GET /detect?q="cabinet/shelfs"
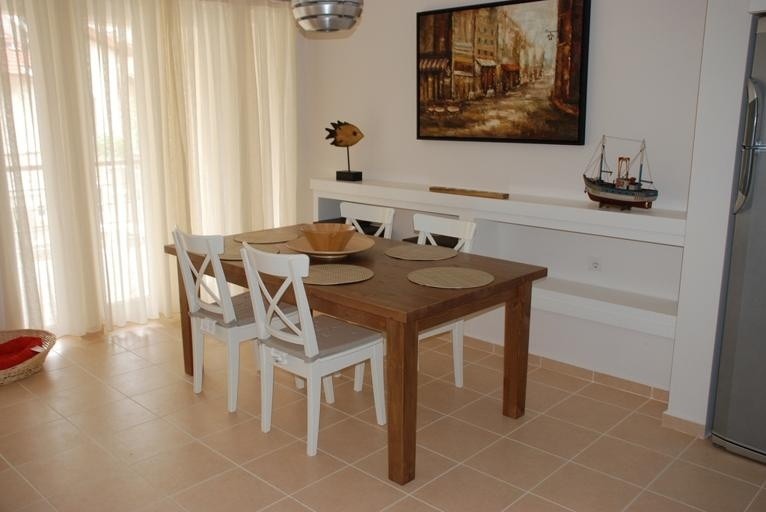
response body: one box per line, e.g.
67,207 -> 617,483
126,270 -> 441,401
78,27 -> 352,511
312,177 -> 686,336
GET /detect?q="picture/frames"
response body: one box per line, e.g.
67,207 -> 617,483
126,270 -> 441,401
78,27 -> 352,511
416,1 -> 593,146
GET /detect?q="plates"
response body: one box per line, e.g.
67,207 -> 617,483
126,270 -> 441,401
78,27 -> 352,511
285,236 -> 375,259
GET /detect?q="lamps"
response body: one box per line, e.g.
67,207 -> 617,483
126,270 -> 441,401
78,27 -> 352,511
292,0 -> 363,34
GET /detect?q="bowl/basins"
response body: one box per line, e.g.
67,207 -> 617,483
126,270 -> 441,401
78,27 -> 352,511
300,223 -> 356,252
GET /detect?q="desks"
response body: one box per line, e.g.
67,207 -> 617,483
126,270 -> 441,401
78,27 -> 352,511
165,225 -> 548,483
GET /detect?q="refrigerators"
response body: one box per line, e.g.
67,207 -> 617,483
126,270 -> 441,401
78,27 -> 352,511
709,14 -> 765,465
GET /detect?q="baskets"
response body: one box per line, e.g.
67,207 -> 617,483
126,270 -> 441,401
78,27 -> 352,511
1,329 -> 57,386
